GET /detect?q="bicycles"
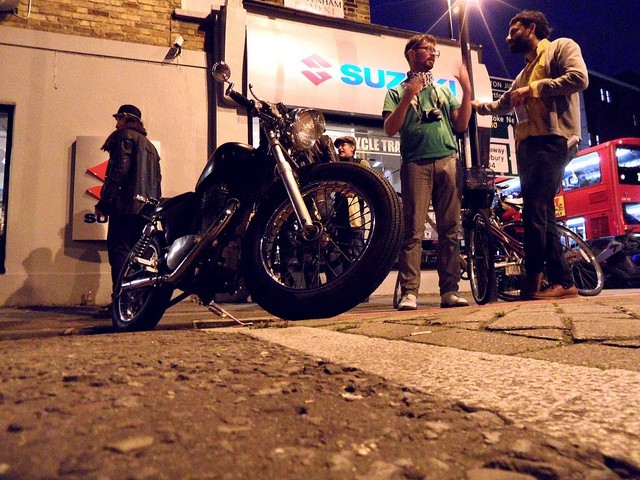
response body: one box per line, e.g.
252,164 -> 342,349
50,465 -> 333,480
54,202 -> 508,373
461,164 -> 603,305
394,191 -> 522,308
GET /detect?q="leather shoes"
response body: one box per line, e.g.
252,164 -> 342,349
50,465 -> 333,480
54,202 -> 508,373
520,272 -> 543,300
440,291 -> 468,307
398,294 -> 417,310
530,284 -> 578,299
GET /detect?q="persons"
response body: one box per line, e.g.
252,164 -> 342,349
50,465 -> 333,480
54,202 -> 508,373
383,35 -> 475,310
335,135 -> 373,304
472,9 -> 590,301
95,104 -> 160,305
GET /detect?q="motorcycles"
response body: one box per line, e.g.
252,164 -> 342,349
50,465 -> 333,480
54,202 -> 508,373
111,60 -> 405,327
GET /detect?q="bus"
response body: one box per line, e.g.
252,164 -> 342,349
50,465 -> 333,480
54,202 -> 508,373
490,137 -> 640,282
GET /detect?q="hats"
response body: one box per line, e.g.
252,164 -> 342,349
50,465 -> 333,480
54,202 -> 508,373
334,136 -> 356,150
113,105 -> 141,121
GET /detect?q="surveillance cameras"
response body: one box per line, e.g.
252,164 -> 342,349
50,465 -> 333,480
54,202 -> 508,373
172,35 -> 186,59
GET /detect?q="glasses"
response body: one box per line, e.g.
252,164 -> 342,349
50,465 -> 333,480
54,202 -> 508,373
413,46 -> 440,56
